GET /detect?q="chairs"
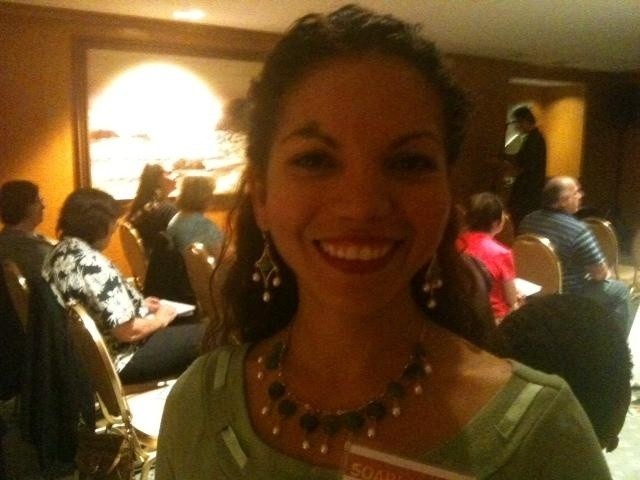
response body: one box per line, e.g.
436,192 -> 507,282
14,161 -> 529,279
3,258 -> 31,332
184,242 -> 216,319
443,208 -> 640,352
66,305 -> 176,480
119,222 -> 149,291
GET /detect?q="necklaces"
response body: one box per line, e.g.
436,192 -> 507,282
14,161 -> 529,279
248,319 -> 437,455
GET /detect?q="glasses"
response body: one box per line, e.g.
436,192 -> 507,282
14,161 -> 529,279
34,198 -> 44,202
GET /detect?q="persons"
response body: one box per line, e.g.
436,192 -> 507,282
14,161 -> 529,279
486,105 -> 549,218
34,187 -> 224,384
399,200 -> 505,360
483,292 -> 636,454
159,173 -> 233,262
121,158 -> 189,255
1,179 -> 75,299
513,174 -> 614,292
452,190 -> 531,327
154,2 -> 613,479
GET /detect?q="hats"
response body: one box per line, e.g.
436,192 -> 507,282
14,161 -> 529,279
466,191 -> 495,223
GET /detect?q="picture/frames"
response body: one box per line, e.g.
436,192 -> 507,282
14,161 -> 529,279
69,36 -> 275,212
